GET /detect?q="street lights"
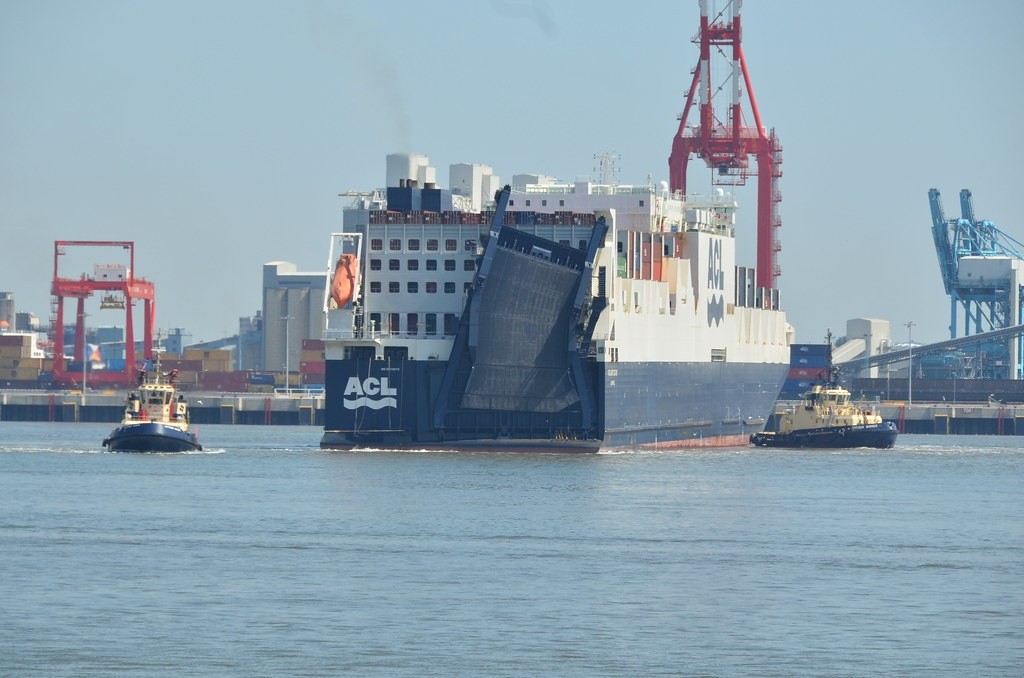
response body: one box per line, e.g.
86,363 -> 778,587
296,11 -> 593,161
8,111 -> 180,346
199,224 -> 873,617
902,321 -> 917,406
280,313 -> 295,394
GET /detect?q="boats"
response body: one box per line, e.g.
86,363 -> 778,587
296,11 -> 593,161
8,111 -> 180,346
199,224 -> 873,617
749,328 -> 899,449
100,328 -> 203,454
330,253 -> 357,309
319,150 -> 793,454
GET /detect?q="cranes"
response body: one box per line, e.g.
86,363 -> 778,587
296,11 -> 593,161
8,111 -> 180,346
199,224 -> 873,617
668,0 -> 784,310
929,186 -> 1024,380
